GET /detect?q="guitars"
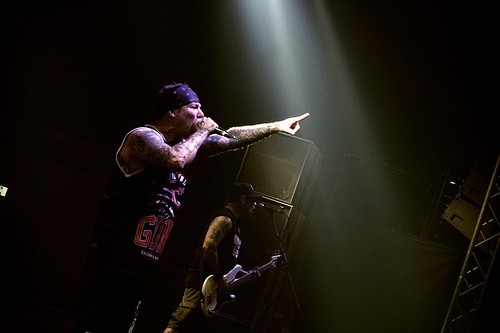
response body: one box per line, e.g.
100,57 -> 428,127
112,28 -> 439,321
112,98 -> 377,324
199,254 -> 280,318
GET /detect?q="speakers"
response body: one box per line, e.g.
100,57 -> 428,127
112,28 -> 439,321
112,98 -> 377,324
192,132 -> 320,333
440,169 -> 500,251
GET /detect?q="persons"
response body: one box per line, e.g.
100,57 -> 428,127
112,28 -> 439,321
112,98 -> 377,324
162,182 -> 263,333
69,83 -> 296,333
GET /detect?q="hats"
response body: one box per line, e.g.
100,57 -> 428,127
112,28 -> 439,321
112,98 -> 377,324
229,182 -> 262,200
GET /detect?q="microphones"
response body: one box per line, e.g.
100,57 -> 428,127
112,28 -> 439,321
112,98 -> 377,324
258,202 -> 284,214
213,127 -> 236,139
